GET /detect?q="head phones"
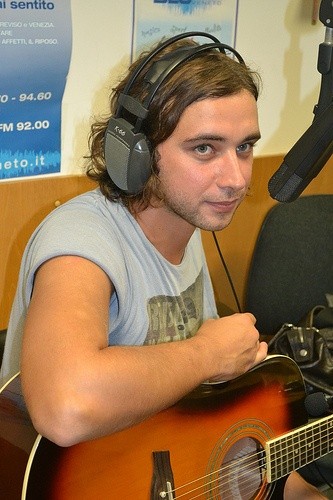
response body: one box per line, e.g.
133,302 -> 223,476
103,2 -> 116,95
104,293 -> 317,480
105,32 -> 245,195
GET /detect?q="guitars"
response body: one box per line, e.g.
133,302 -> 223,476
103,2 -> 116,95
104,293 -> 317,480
0,354 -> 333,500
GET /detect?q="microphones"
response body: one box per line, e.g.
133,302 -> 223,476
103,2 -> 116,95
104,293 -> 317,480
305,392 -> 333,416
267,99 -> 333,203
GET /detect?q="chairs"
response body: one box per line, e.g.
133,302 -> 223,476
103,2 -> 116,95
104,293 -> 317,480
241,194 -> 333,336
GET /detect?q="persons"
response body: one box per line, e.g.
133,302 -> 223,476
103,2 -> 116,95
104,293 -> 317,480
0,32 -> 329,500
